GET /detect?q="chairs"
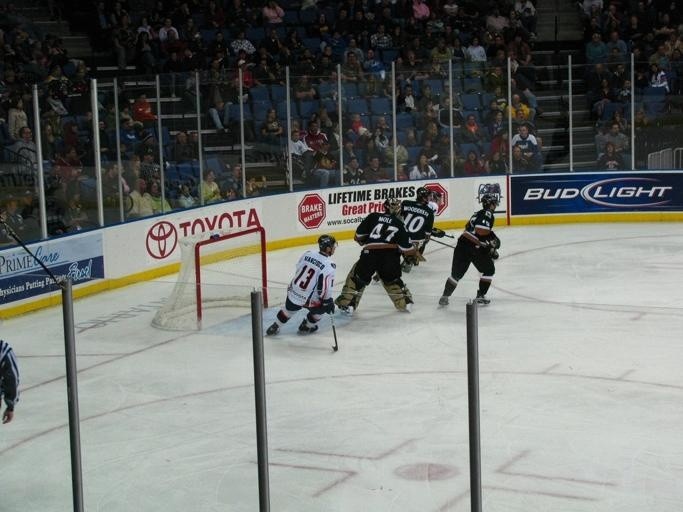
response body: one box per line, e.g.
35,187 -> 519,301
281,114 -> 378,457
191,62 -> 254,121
1,4 -> 681,248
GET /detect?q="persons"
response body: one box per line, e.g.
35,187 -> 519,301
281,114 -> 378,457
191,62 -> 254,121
266,234 -> 335,337
579,1 -> 683,171
439,194 -> 500,308
0,339 -> 21,424
334,198 -> 414,313
0,1 -> 539,247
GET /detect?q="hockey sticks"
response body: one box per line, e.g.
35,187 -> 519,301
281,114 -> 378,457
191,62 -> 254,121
329,312 -> 338,350
445,234 -> 454,239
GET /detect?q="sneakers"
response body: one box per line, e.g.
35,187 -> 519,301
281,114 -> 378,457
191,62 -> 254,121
340,305 -> 354,314
439,295 -> 449,305
405,301 -> 412,310
476,290 -> 490,304
298,317 -> 318,332
266,321 -> 280,335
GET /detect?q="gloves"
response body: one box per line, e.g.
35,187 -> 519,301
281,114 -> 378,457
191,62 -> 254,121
486,231 -> 501,250
320,297 -> 335,315
492,249 -> 499,260
430,227 -> 446,239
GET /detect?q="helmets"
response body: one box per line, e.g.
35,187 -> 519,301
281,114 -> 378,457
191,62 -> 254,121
317,234 -> 335,249
383,196 -> 402,217
429,190 -> 442,203
480,194 -> 498,204
416,187 -> 429,200
